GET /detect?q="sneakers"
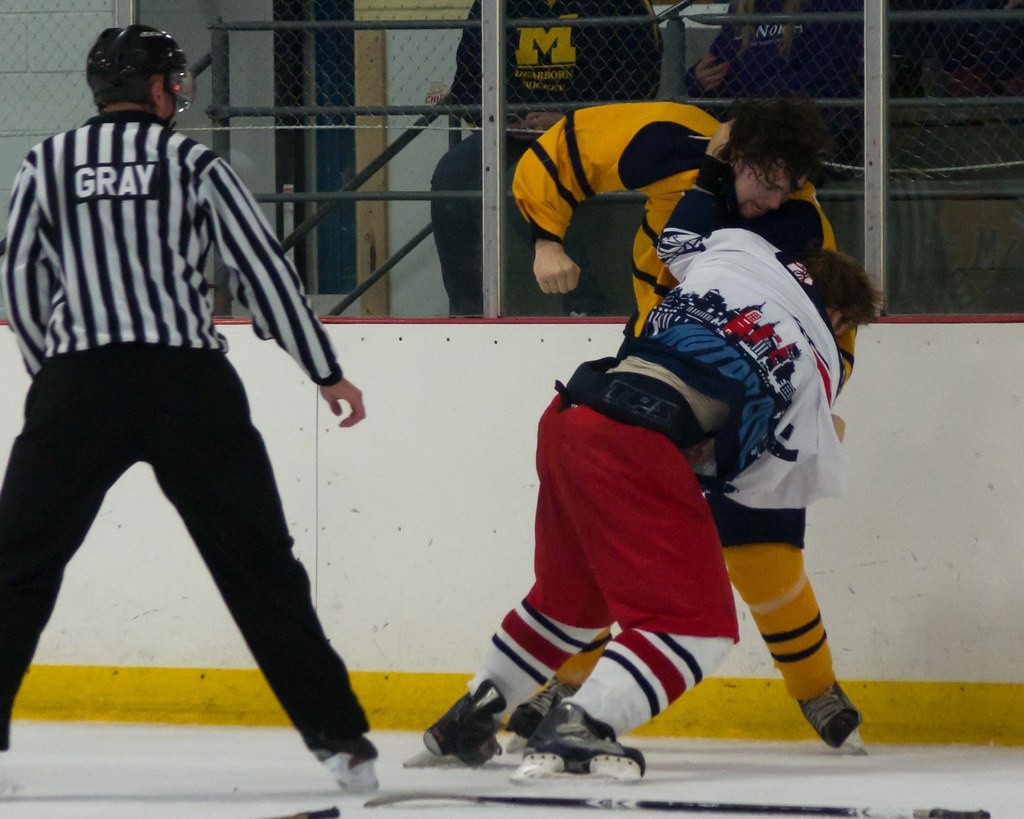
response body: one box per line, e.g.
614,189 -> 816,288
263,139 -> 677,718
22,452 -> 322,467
510,703 -> 645,787
402,680 -> 508,769
800,678 -> 867,752
331,741 -> 381,794
508,677 -> 583,737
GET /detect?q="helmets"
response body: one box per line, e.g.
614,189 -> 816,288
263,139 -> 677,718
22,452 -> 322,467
86,24 -> 197,111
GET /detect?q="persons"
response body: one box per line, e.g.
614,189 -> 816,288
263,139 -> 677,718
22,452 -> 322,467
1,18 -> 405,808
417,220 -> 884,788
427,1 -> 663,318
510,81 -> 858,779
677,0 -> 876,228
